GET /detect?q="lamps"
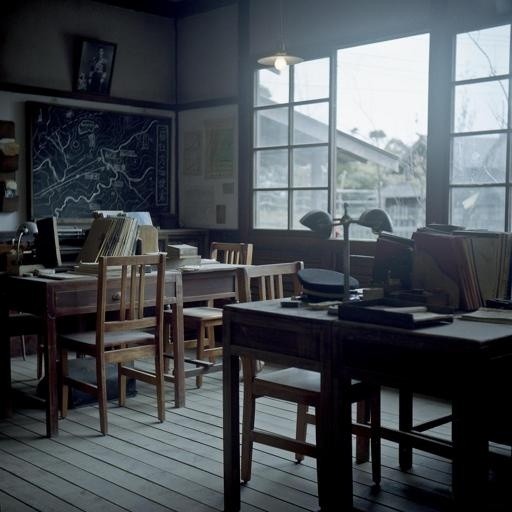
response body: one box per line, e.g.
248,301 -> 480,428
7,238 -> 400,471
257,0 -> 304,72
299,201 -> 393,311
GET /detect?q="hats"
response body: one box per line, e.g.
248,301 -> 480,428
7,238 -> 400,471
297,268 -> 359,302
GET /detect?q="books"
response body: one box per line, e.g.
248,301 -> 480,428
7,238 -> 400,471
76,214 -> 142,279
371,221 -> 511,313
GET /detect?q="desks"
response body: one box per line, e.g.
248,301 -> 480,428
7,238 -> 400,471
221,287 -> 511,511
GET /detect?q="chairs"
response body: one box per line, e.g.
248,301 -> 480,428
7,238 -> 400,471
237,260 -> 369,484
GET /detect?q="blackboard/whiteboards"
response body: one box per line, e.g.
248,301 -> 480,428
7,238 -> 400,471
25,100 -> 173,226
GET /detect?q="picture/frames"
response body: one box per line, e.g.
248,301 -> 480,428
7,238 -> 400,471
72,36 -> 117,97
